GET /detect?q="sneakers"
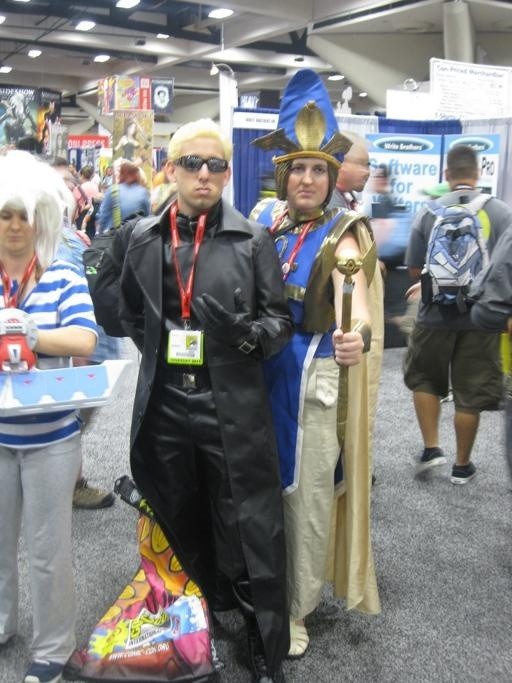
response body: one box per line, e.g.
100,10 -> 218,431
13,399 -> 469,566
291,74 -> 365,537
414,446 -> 446,477
23,659 -> 63,683
449,462 -> 477,485
73,478 -> 115,508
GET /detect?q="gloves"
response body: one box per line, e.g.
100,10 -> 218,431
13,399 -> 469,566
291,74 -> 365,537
191,289 -> 261,356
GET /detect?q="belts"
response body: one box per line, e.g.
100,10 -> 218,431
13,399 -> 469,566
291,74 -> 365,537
180,373 -> 197,390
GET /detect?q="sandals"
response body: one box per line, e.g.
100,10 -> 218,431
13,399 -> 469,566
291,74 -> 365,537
288,616 -> 309,657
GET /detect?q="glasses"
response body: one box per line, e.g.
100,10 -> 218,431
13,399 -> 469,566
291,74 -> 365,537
174,155 -> 228,173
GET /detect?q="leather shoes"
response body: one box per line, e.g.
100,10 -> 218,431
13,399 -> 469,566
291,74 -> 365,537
248,653 -> 286,682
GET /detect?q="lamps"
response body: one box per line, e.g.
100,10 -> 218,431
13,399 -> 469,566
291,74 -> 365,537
403,77 -> 420,92
210,63 -> 235,78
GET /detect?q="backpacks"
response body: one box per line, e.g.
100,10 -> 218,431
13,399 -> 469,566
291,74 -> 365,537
81,208 -> 146,337
417,193 -> 497,314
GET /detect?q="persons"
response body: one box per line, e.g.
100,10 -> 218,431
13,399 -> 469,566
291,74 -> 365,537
407,146 -> 512,486
113,115 -> 296,682
327,130 -> 388,216
21,137 -> 177,511
0,148 -> 101,683
245,156 -> 385,661
